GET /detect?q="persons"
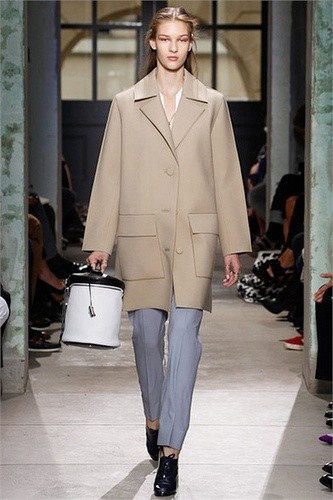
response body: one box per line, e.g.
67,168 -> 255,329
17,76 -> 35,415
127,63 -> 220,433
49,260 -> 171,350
81,9 -> 253,497
246,144 -> 306,350
26,191 -> 82,353
308,272 -> 333,395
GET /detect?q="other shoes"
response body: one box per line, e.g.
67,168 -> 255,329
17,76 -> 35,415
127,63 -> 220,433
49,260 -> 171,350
252,264 -> 275,286
324,411 -> 333,418
285,335 -> 304,351
319,433 -> 333,445
28,337 -> 62,352
38,276 -> 66,295
260,297 -> 284,314
327,402 -> 332,409
47,261 -> 86,279
326,419 -> 333,427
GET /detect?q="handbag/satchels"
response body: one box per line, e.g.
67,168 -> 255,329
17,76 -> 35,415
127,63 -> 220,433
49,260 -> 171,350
59,268 -> 125,350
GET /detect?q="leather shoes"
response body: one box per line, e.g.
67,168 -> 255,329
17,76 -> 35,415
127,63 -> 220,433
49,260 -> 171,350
319,473 -> 333,489
146,426 -> 159,462
154,453 -> 179,497
322,461 -> 333,473
